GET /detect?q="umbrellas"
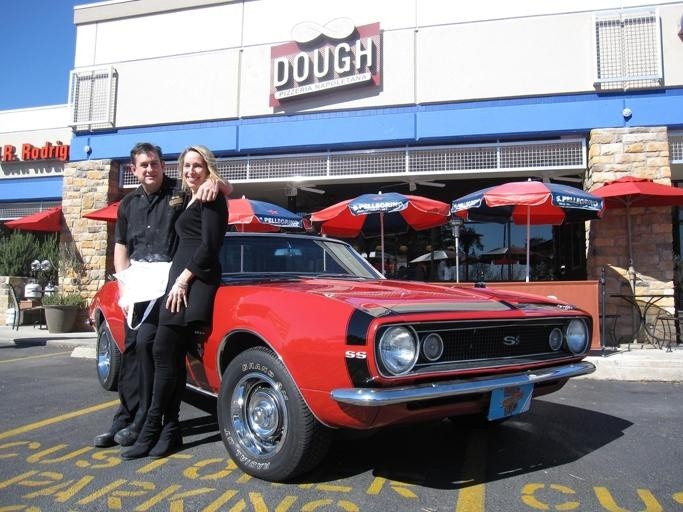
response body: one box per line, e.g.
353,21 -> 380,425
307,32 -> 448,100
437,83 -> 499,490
227,194 -> 307,272
408,248 -> 480,279
302,190 -> 451,279
587,176 -> 683,348
478,246 -> 552,265
448,178 -> 606,282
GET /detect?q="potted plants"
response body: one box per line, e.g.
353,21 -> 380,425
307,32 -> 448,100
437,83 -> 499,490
41,293 -> 84,334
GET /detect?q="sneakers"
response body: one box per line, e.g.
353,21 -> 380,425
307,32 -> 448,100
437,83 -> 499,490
114,425 -> 138,444
94,432 -> 115,446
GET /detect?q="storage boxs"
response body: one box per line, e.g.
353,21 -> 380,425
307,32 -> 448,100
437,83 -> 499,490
20,301 -> 40,309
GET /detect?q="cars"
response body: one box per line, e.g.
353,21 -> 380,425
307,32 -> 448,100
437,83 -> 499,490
86,230 -> 598,484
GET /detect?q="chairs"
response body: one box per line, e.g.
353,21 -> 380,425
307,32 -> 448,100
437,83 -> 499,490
6,284 -> 44,331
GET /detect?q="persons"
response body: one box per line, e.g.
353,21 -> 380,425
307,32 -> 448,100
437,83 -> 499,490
120,145 -> 229,461
94,142 -> 234,448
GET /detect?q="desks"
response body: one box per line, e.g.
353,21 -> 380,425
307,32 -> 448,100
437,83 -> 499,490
610,295 -> 674,350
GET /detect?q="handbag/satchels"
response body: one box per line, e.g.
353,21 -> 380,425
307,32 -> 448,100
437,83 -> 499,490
110,259 -> 173,306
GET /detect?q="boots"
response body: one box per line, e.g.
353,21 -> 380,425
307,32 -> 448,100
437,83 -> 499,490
121,425 -> 161,459
149,420 -> 183,457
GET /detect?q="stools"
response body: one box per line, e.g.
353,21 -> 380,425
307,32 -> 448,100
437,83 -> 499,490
599,314 -> 621,348
657,317 -> 683,352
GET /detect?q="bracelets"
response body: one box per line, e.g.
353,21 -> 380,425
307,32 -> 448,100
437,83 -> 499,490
176,277 -> 189,289
